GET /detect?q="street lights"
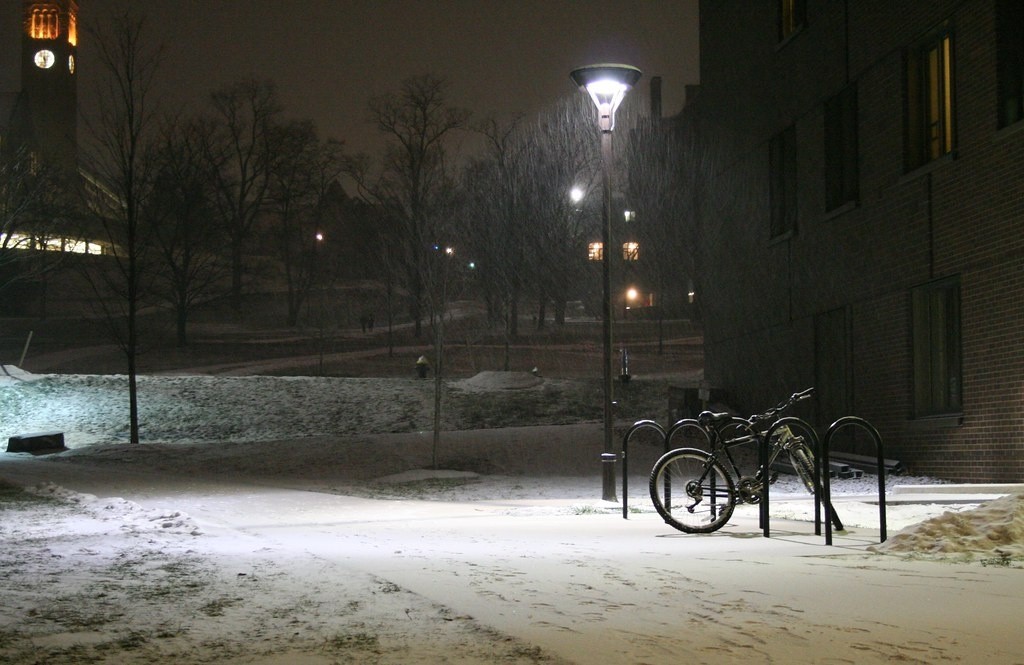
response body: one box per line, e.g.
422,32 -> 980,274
567,61 -> 647,504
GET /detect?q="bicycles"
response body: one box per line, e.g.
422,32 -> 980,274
649,385 -> 846,536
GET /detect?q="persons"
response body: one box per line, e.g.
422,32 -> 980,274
359,314 -> 374,332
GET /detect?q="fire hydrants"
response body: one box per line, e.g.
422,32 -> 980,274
414,356 -> 431,379
